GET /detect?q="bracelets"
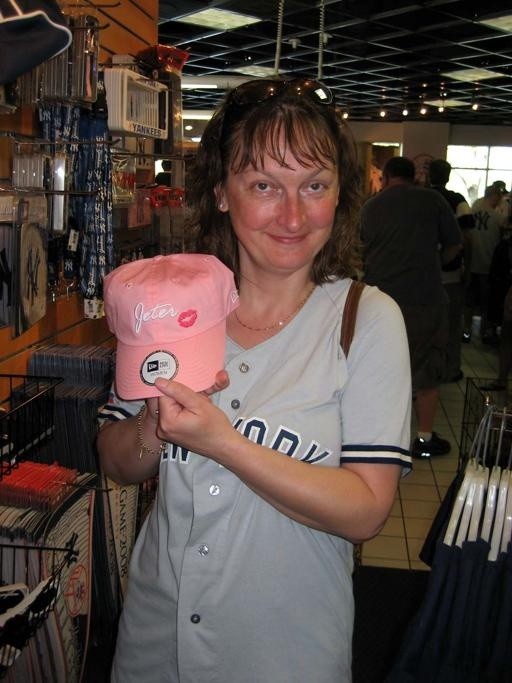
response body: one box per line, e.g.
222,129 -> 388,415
137,402 -> 168,460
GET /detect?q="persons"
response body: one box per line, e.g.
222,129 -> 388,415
428,157 -> 473,383
93,77 -> 414,682
355,154 -> 464,461
470,180 -> 512,392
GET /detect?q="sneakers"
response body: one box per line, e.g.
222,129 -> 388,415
413,432 -> 450,458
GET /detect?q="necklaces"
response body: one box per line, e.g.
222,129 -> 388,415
234,283 -> 315,331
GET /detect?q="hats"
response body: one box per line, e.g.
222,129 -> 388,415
103,253 -> 240,401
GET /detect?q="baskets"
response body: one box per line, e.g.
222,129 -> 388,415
0,544 -> 74,678
0,375 -> 64,481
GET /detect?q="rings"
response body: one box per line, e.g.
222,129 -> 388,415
156,410 -> 161,415
